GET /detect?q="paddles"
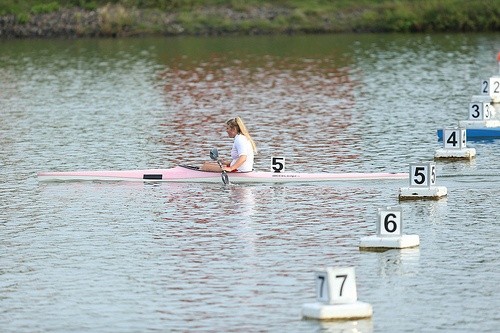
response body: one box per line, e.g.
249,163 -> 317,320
210,149 -> 230,185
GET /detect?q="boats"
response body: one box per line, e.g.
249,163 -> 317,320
36,165 -> 410,184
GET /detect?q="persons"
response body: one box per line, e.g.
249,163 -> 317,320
202,117 -> 257,172
